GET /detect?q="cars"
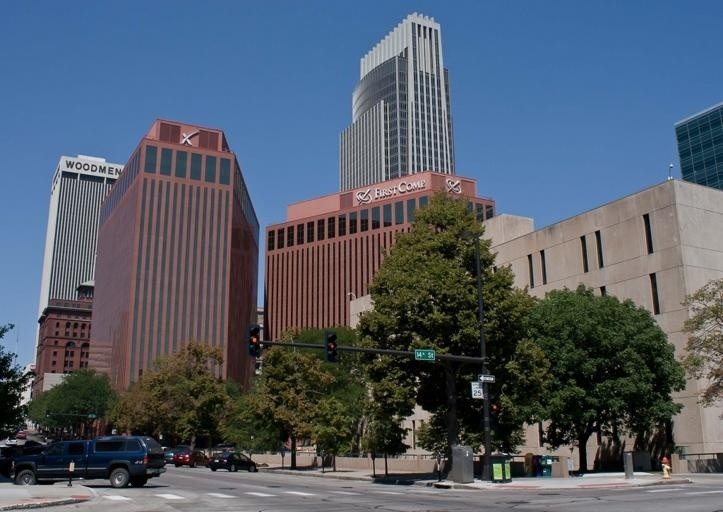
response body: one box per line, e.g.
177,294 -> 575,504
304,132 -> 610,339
4,422 -> 74,447
162,445 -> 257,473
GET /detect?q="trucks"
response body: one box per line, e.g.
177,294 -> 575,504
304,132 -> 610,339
8,435 -> 168,489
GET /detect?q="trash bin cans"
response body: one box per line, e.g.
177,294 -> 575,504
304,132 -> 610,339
533,455 -> 551,476
491,456 -> 512,482
452,446 -> 474,483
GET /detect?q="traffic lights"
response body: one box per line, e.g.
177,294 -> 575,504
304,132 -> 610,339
489,395 -> 499,414
324,331 -> 338,362
247,322 -> 260,357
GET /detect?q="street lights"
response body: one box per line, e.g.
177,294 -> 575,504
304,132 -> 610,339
459,230 -> 494,481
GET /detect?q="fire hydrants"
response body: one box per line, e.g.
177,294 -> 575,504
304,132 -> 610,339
660,456 -> 673,479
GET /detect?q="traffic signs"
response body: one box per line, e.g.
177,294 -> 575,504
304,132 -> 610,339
471,381 -> 484,400
414,349 -> 435,361
477,374 -> 496,384
